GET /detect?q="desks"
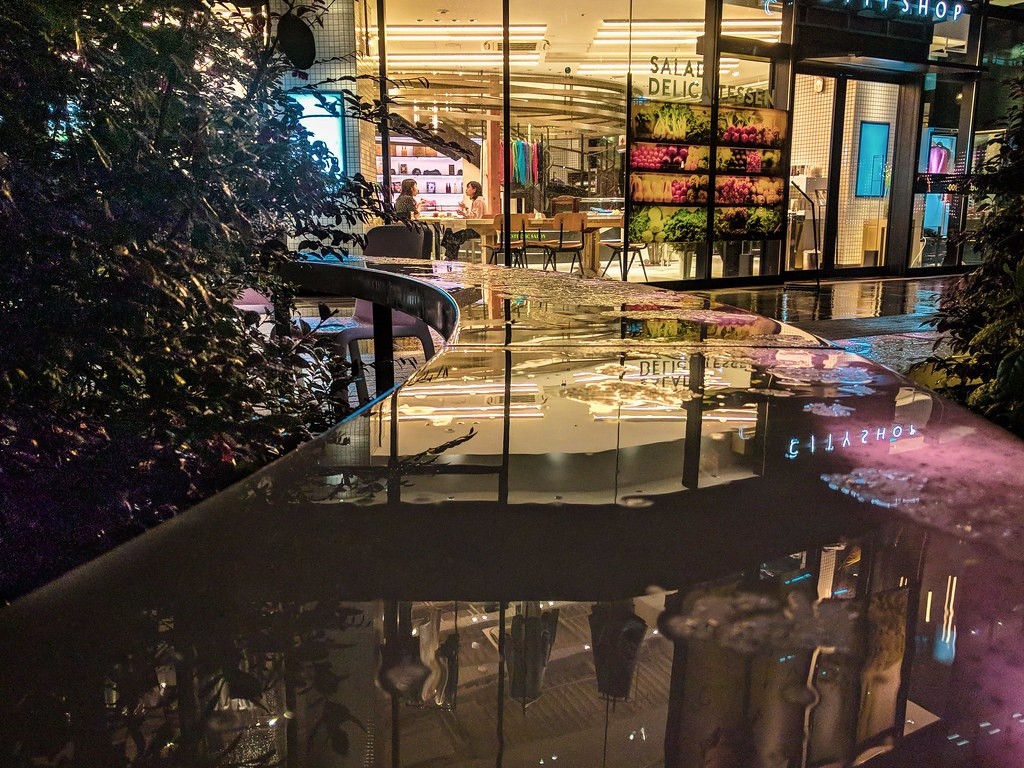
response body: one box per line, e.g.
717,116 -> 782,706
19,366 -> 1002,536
416,217 -> 459,260
448,214 -> 623,273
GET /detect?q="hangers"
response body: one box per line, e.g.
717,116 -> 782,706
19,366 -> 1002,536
510,135 -> 541,143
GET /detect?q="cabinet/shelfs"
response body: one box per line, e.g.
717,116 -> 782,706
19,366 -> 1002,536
375,137 -> 482,217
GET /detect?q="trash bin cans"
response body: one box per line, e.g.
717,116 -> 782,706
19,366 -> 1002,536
802,249 -> 821,270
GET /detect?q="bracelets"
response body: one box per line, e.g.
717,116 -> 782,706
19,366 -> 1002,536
466,208 -> 468,211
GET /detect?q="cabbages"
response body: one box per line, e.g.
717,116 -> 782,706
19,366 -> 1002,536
641,220 -> 666,243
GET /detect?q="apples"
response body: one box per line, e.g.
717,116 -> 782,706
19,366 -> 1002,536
630,146 -> 666,169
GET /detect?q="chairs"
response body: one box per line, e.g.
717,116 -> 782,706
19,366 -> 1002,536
289,225 -> 435,407
478,213 -> 533,268
601,212 -> 648,282
518,214 -> 555,271
542,213 -> 588,275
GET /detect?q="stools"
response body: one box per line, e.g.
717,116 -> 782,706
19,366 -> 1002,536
466,239 -> 480,263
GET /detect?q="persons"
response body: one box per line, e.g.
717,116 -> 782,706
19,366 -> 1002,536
457,181 -> 486,219
551,172 -> 565,185
394,179 -> 426,218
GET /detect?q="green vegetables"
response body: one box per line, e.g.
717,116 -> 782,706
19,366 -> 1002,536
667,207 -> 781,240
635,101 -> 711,143
627,205 -> 653,242
